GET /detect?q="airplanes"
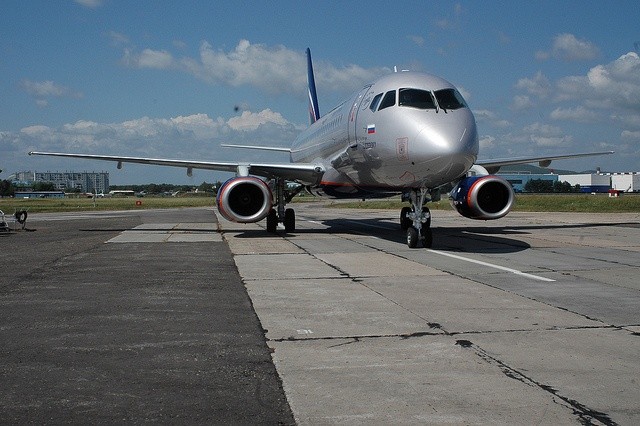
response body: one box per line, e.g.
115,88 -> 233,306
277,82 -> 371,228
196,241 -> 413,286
29,46 -> 615,248
